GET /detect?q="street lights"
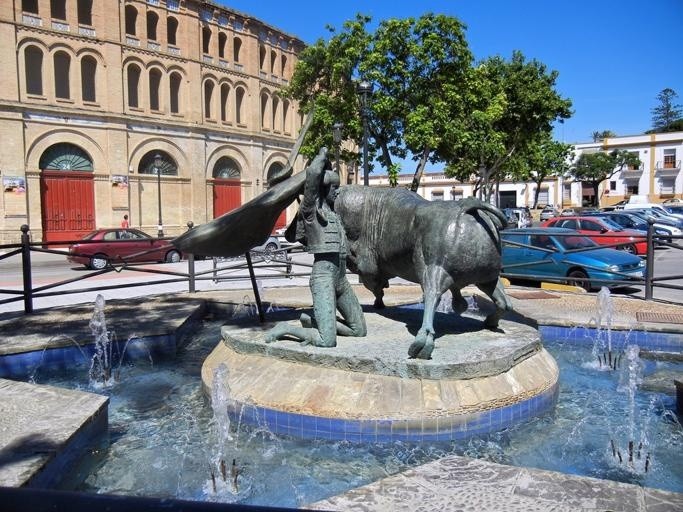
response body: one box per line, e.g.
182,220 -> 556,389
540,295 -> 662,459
154,153 -> 165,239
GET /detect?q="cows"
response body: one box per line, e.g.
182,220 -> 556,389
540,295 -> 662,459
283,180 -> 510,361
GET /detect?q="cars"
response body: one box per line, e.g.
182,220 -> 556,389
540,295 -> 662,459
68,227 -> 185,269
249,234 -> 281,255
539,198 -> 683,256
275,223 -> 309,253
496,226 -> 647,295
509,207 -> 533,229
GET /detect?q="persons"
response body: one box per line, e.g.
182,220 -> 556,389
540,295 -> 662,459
265,147 -> 368,347
121,215 -> 129,228
